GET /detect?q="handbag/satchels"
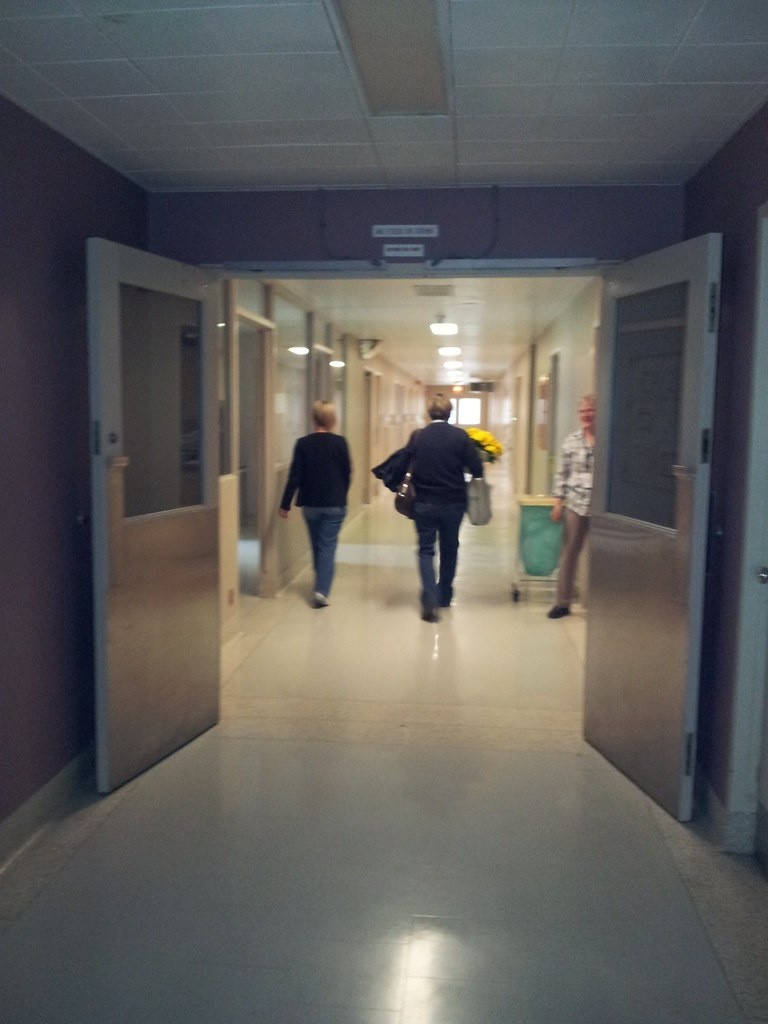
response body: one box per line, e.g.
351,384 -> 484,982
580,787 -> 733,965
396,477 -> 418,519
466,479 -> 492,526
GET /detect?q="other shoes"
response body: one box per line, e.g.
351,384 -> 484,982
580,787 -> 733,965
548,605 -> 570,619
422,596 -> 436,620
313,591 -> 330,606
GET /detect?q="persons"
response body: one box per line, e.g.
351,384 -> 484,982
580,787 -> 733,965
278,400 -> 353,607
371,393 -> 490,622
545,393 -> 595,619
